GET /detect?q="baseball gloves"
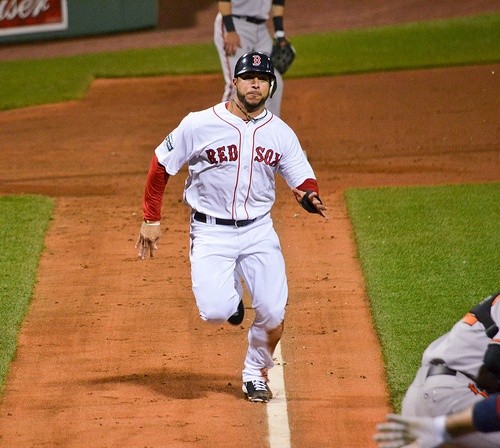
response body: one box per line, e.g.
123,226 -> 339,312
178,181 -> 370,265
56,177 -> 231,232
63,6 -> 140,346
269,37 -> 298,74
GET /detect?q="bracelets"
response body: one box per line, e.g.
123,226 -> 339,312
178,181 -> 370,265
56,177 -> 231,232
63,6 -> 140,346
223,15 -> 236,32
273,16 -> 283,32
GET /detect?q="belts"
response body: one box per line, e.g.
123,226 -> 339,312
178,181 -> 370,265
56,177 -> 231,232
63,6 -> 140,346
232,14 -> 267,25
426,365 -> 458,376
193,211 -> 271,228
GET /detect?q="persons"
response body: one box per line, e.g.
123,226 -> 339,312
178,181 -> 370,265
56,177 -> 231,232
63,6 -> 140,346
134,51 -> 328,402
213,0 -> 295,118
372,290 -> 500,448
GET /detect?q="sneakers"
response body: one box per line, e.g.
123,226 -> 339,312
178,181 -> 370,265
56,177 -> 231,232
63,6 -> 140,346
226,299 -> 245,326
241,380 -> 273,403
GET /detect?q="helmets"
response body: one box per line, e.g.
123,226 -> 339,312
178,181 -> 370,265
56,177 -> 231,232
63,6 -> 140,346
234,51 -> 274,77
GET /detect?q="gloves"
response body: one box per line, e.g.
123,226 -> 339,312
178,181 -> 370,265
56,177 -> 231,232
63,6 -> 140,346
372,413 -> 448,448
